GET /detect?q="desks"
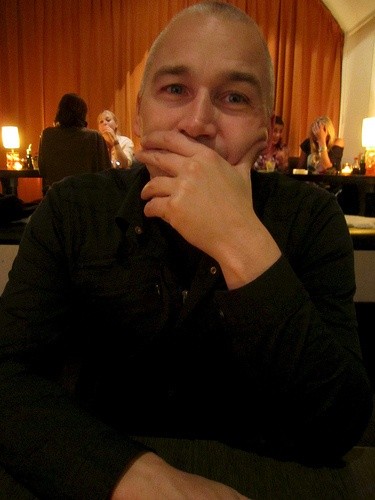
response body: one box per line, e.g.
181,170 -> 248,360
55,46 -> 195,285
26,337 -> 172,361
256,170 -> 375,219
0,435 -> 375,500
0,156 -> 42,198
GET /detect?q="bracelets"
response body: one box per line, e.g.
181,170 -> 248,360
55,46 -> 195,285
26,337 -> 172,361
318,147 -> 327,152
110,140 -> 118,148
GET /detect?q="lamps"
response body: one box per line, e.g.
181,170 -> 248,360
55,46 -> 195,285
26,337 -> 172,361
2,125 -> 20,161
361,117 -> 375,152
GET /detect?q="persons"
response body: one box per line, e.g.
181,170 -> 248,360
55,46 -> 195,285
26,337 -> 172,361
0,3 -> 357,500
38,93 -> 112,198
299,116 -> 343,175
253,116 -> 288,171
97,110 -> 134,169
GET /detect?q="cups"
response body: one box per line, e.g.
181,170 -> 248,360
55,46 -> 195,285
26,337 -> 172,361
7,152 -> 19,169
113,160 -> 122,169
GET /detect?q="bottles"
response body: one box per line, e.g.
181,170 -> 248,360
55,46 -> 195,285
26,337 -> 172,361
23,148 -> 33,170
352,157 -> 366,175
